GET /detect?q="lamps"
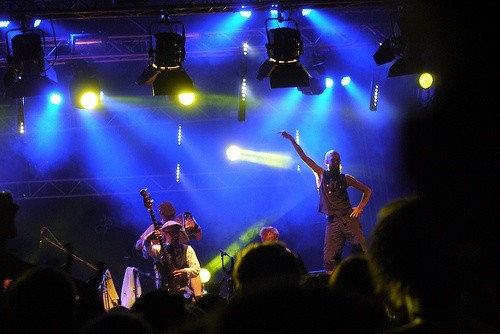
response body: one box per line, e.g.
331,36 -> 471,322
373,11 -> 431,77
299,66 -> 326,94
6,28 -> 52,98
136,20 -> 194,97
69,72 -> 102,109
257,17 -> 312,89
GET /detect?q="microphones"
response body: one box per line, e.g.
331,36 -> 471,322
40,228 -> 44,247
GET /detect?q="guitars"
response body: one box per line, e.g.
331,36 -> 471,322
139,187 -> 191,293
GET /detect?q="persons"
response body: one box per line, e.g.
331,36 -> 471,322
277,130 -> 372,276
0,190 -> 500,334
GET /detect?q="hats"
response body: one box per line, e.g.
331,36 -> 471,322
161,220 -> 182,231
